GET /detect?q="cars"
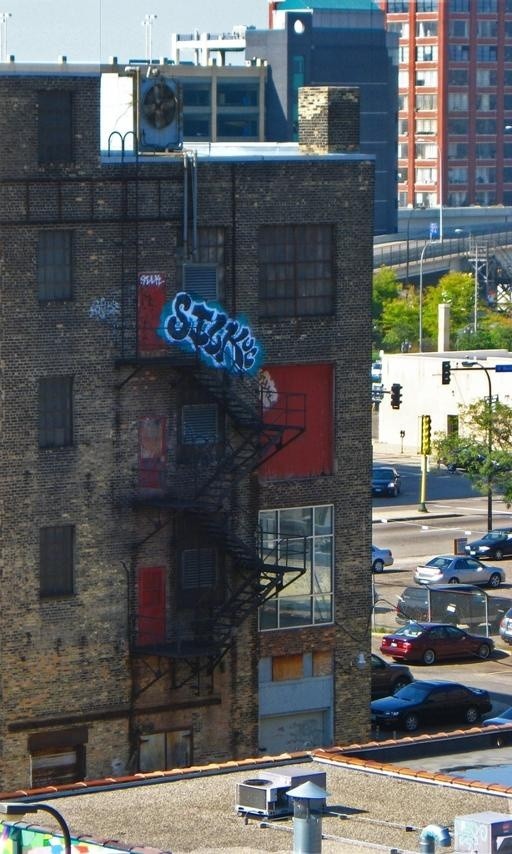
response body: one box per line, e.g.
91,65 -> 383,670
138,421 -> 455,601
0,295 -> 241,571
437,448 -> 512,472
371,528 -> 512,731
373,467 -> 401,497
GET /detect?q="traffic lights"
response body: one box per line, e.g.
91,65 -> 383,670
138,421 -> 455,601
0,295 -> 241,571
442,361 -> 450,384
423,416 -> 432,454
391,384 -> 402,409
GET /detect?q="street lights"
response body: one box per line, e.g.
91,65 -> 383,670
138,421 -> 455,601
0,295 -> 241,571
0,10 -> 12,63
505,126 -> 512,129
462,362 -> 492,539
420,241 -> 437,353
142,13 -> 158,65
455,229 -> 478,333
406,207 -> 426,282
0,802 -> 71,854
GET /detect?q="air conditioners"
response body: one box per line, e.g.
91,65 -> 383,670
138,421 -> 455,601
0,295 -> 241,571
232,777 -> 293,819
